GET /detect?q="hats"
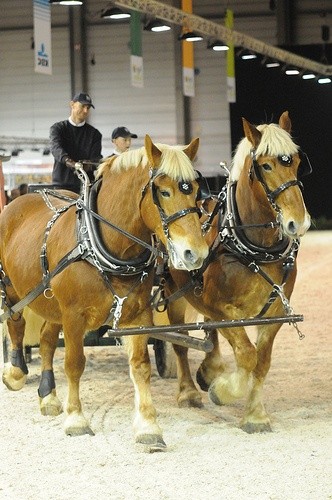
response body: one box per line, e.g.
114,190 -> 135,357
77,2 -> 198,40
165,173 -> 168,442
112,126 -> 137,139
73,93 -> 95,109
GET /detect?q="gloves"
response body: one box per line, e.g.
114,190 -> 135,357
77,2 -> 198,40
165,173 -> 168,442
64,157 -> 76,167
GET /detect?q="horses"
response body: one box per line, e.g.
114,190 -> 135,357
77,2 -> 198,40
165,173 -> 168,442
0,111 -> 312,455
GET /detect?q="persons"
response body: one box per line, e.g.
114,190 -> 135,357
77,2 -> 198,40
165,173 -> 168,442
50,92 -> 102,189
107,126 -> 138,158
